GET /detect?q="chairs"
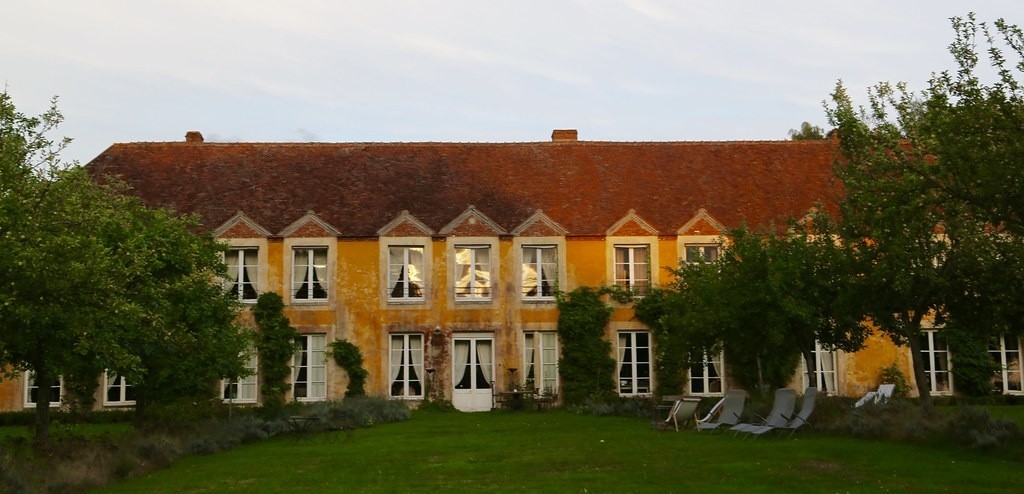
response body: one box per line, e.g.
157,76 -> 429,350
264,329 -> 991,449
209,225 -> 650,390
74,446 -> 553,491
696,389 -> 746,434
651,395 -> 703,432
852,384 -> 896,408
322,426 -> 357,444
777,387 -> 818,442
728,389 -> 796,441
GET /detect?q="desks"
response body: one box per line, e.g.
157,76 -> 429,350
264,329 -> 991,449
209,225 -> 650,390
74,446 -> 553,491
1002,390 -> 1024,407
285,416 -> 321,446
